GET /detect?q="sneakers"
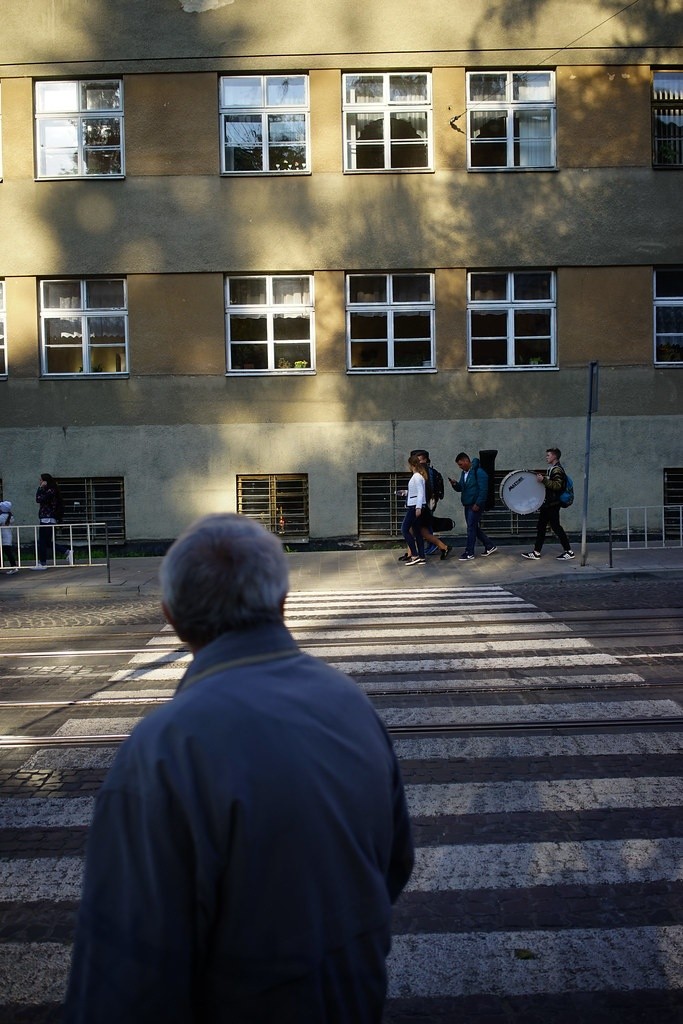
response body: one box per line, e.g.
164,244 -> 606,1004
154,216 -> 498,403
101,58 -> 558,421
399,553 -> 411,562
416,559 -> 426,564
426,544 -> 438,555
521,551 -> 541,559
405,556 -> 420,565
440,546 -> 452,560
458,551 -> 474,560
481,544 -> 497,556
424,548 -> 438,555
556,551 -> 575,560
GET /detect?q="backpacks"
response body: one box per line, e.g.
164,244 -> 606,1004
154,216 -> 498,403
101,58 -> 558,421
429,466 -> 444,500
43,485 -> 65,519
548,466 -> 574,508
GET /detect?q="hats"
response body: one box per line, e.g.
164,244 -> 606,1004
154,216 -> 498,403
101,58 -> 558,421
0,501 -> 12,512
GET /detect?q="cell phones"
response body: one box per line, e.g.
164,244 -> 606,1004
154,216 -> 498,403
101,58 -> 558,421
395,491 -> 404,496
448,478 -> 452,482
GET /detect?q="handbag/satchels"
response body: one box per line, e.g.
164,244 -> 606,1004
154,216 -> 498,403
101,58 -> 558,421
475,450 -> 499,513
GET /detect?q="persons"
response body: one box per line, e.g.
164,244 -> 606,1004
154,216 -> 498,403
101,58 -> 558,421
30,473 -> 75,570
520,448 -> 575,560
63,508 -> 414,1024
0,501 -> 19,574
450,453 -> 498,560
397,450 -> 453,565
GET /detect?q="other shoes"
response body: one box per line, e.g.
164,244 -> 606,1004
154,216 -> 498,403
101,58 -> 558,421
67,551 -> 71,564
7,568 -> 19,575
31,563 -> 47,570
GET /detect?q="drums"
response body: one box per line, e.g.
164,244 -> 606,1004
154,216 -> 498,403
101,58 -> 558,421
499,469 -> 547,516
